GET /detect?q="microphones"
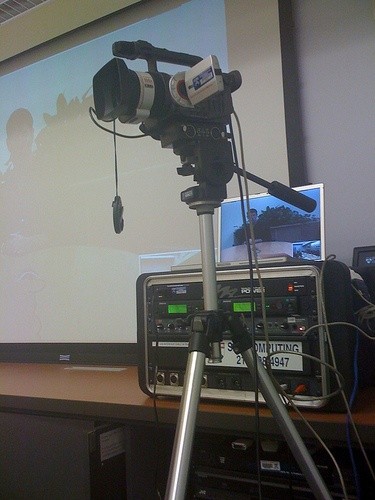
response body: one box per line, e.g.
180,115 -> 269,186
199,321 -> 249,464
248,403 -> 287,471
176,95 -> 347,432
235,166 -> 317,213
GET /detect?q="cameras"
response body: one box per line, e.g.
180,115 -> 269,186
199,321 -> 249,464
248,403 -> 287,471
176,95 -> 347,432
87,35 -> 244,234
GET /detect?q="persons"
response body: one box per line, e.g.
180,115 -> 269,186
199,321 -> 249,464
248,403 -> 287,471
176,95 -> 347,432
242,208 -> 271,244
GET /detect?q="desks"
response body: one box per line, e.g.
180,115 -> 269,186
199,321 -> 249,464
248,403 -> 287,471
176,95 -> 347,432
0,361 -> 375,500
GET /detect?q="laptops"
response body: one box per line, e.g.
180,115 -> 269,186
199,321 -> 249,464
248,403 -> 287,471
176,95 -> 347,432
168,183 -> 325,271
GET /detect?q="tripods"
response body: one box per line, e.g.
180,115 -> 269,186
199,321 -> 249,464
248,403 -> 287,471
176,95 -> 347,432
162,181 -> 334,500
